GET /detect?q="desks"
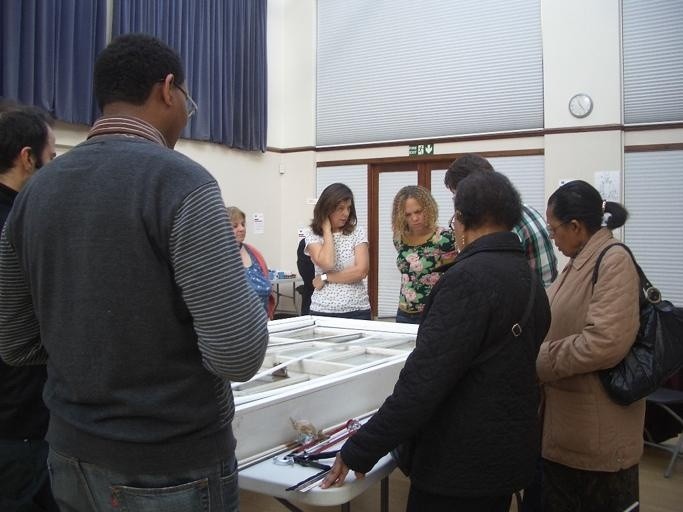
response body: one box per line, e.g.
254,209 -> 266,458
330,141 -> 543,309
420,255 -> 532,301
265,271 -> 304,316
232,410 -> 397,510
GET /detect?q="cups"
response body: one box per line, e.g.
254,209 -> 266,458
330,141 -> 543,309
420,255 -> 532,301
269,272 -> 273,280
278,272 -> 284,279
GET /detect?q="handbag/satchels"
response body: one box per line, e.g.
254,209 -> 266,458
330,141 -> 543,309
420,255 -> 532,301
390,425 -> 427,477
600,299 -> 683,406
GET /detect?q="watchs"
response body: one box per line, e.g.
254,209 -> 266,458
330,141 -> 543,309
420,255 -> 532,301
321,272 -> 328,286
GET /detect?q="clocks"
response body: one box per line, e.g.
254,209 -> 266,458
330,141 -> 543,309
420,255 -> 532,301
569,91 -> 594,118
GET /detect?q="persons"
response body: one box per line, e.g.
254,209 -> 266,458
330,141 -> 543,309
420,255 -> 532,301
225,207 -> 277,322
320,171 -> 552,511
303,183 -> 374,320
443,154 -> 560,287
533,181 -> 645,511
0,35 -> 268,512
391,186 -> 459,324
0,107 -> 58,510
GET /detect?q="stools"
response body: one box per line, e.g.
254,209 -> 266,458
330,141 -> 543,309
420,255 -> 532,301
643,385 -> 682,477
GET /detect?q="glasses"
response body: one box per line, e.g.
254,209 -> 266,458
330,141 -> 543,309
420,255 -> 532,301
448,210 -> 462,231
150,75 -> 198,118
546,219 -> 572,238
